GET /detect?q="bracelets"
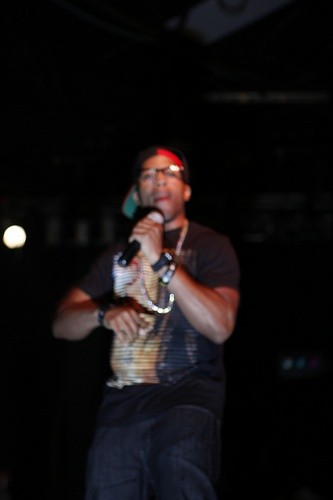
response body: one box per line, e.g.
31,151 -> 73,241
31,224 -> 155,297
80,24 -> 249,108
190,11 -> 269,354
98,306 -> 109,327
158,262 -> 178,287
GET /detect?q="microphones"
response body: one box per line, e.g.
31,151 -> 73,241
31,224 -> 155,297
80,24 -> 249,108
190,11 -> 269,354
117,211 -> 164,267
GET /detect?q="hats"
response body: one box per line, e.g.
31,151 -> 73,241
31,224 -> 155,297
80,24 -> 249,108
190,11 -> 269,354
135,143 -> 189,183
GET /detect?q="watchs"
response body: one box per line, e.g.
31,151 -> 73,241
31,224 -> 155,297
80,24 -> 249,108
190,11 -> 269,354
151,252 -> 174,272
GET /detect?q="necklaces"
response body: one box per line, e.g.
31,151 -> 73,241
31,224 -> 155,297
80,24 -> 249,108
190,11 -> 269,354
139,220 -> 188,314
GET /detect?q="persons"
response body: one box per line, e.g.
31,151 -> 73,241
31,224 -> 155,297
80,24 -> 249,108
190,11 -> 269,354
51,148 -> 241,500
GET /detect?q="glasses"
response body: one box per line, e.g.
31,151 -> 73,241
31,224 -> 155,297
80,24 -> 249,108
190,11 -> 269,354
140,168 -> 184,184
